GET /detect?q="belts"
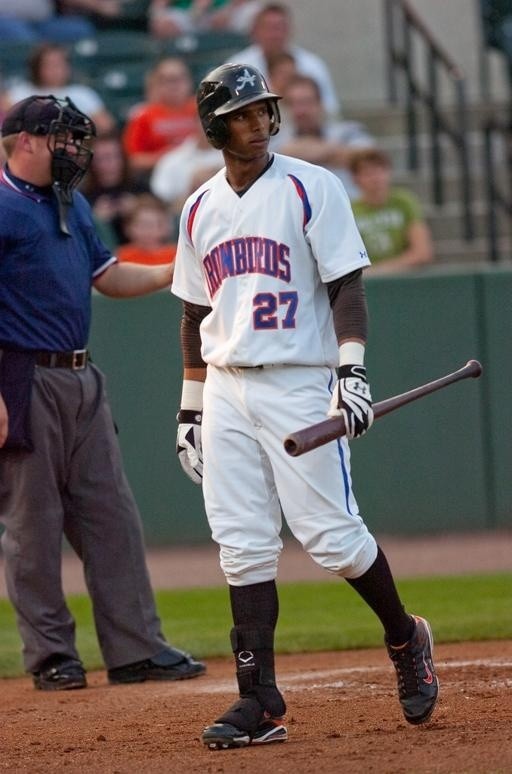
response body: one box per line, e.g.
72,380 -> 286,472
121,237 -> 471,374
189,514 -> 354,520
37,347 -> 92,372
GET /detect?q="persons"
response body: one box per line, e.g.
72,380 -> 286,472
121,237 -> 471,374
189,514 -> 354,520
176,64 -> 439,750
1,99 -> 208,690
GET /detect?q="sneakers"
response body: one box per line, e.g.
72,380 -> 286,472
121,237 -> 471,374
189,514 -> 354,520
202,693 -> 287,747
33,657 -> 87,690
106,650 -> 208,685
384,614 -> 438,725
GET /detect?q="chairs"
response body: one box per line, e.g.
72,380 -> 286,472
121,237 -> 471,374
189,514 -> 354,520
1,1 -> 255,250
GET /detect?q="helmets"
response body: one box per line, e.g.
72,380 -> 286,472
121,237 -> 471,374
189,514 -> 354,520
194,60 -> 282,151
3,93 -> 97,204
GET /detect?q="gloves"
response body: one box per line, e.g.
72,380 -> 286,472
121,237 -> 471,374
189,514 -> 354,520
327,362 -> 374,442
174,407 -> 203,484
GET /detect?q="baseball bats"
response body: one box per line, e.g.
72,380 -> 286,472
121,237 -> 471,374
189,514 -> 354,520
284,360 -> 483,456
